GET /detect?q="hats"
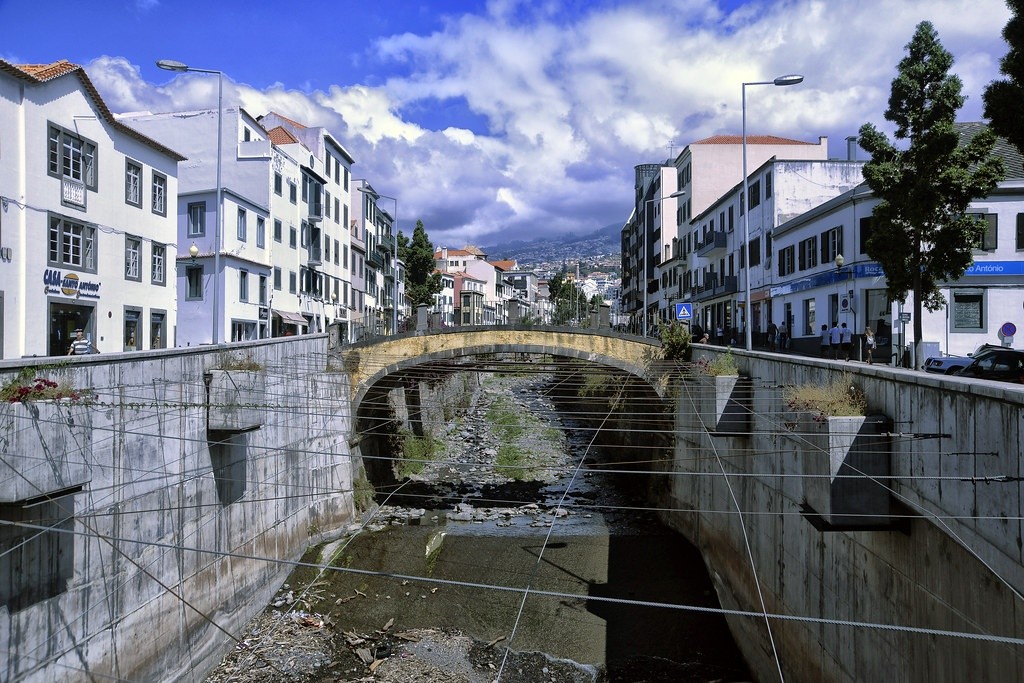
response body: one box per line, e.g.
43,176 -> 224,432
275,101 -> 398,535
77,332 -> 82,336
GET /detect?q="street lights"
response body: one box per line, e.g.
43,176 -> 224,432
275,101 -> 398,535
743,74 -> 804,350
643,190 -> 685,338
157,59 -> 223,346
358,188 -> 398,335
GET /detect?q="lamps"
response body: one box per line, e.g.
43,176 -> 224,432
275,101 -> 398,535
834,254 -> 856,275
176,241 -> 199,267
323,291 -> 337,308
663,291 -> 678,300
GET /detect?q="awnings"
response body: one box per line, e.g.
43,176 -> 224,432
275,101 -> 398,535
274,310 -> 308,326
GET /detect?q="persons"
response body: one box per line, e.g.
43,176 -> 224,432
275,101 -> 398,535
67,332 -> 100,356
816,322 -> 853,361
864,327 -> 875,363
766,320 -> 778,350
699,333 -> 709,344
777,322 -> 788,350
717,324 -> 725,345
729,338 -> 736,345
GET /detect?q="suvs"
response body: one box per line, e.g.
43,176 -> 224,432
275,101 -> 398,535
691,325 -> 704,343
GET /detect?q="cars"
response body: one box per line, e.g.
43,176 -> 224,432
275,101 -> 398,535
953,350 -> 1024,385
923,351 -> 1022,376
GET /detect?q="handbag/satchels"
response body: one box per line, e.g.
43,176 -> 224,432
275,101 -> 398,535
775,336 -> 779,344
873,339 -> 876,349
785,337 -> 790,348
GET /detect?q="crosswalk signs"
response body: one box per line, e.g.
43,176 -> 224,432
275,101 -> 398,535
675,303 -> 692,319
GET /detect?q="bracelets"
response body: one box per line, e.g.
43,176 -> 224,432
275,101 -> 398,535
99,352 -> 100,354
